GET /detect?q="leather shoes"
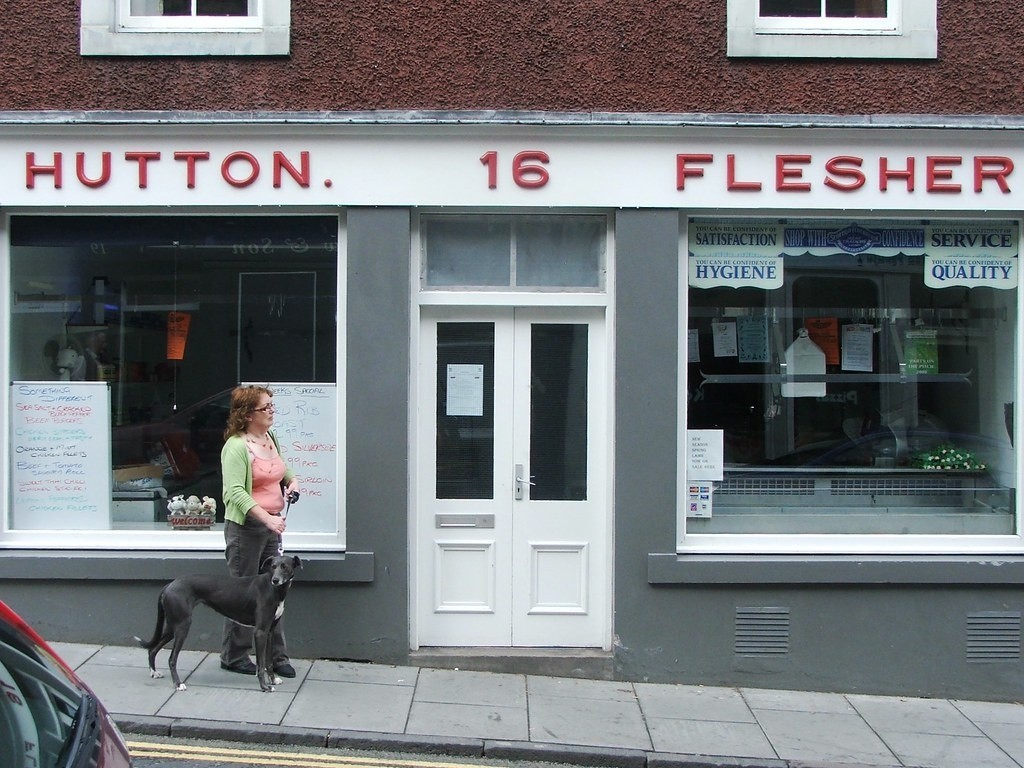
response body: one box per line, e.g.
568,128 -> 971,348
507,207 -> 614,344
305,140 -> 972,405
274,663 -> 296,678
221,656 -> 256,676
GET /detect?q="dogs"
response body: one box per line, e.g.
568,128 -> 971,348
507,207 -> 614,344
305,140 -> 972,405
132,554 -> 303,692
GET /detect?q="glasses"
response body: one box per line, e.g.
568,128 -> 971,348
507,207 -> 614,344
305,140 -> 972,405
252,402 -> 274,412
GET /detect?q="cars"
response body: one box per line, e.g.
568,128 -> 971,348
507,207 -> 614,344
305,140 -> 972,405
111,387 -> 238,466
759,425 -> 1015,469
0,601 -> 134,768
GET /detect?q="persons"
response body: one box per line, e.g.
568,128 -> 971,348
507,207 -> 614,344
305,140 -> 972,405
86,332 -> 109,368
220,385 -> 301,679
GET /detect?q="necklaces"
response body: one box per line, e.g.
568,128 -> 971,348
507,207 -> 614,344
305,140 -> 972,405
247,433 -> 272,450
